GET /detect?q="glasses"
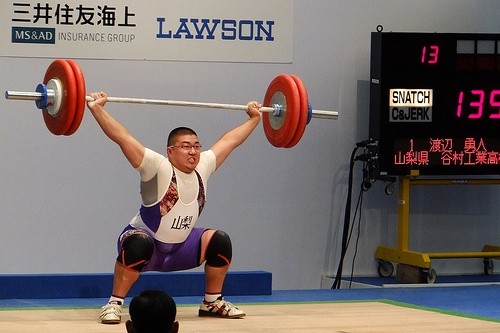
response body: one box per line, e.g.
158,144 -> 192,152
170,144 -> 203,150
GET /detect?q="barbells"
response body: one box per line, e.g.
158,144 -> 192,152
4,57 -> 340,148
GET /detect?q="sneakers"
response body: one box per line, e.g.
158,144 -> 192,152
199,295 -> 246,318
99,300 -> 122,323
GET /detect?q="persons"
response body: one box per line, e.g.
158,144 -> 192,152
87,91 -> 263,324
125,289 -> 179,333
